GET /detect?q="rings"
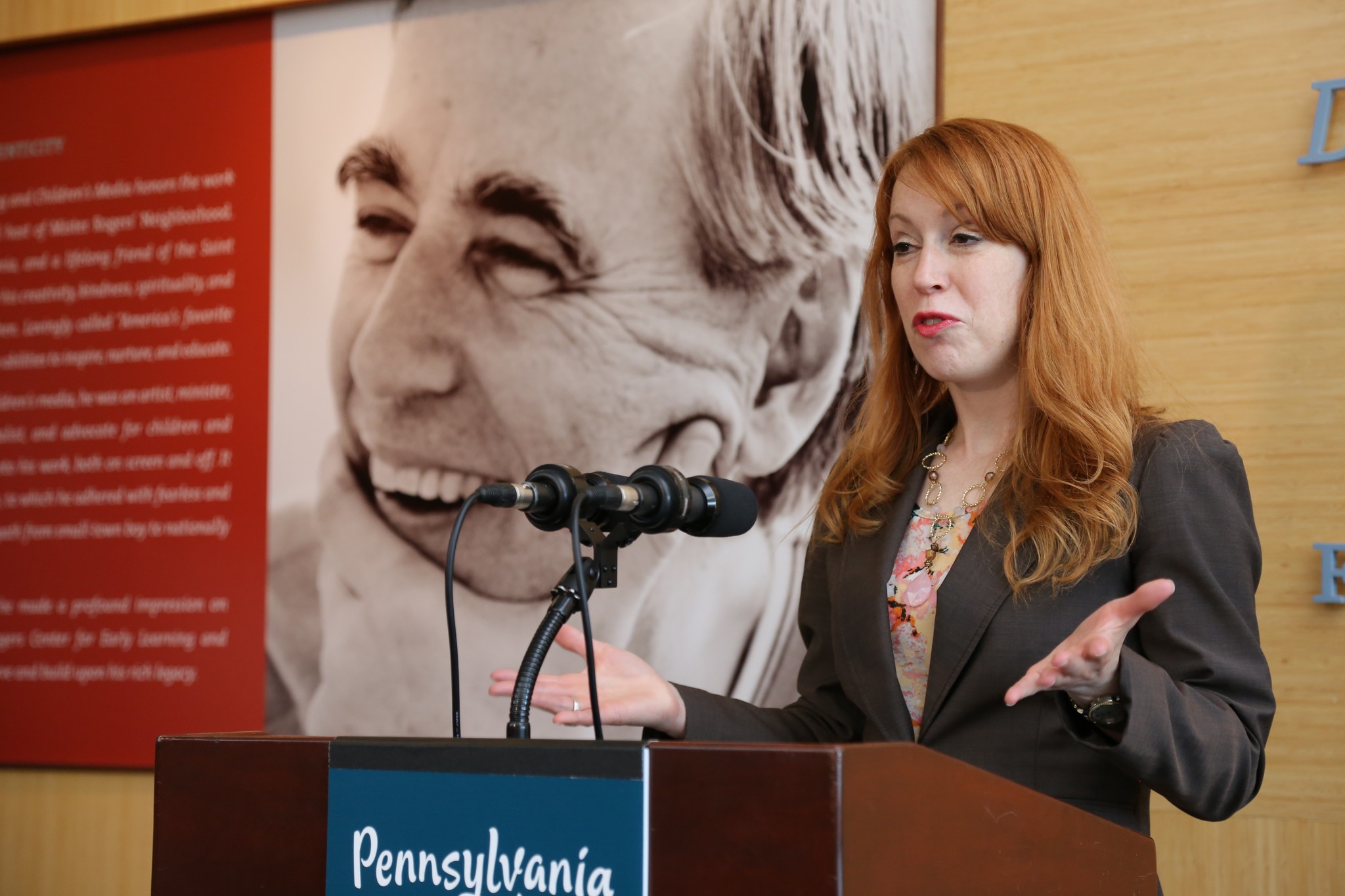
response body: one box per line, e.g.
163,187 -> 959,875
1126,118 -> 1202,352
572,697 -> 579,711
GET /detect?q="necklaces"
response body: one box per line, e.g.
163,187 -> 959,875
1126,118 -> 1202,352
921,421 -> 1016,571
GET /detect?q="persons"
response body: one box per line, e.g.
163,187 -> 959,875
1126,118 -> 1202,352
263,0 -> 926,742
491,119 -> 1278,836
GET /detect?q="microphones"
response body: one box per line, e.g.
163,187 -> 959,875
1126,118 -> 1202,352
586,475 -> 758,537
481,462 -> 628,532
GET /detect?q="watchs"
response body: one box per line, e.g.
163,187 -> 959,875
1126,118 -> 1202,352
1068,694 -> 1132,731
